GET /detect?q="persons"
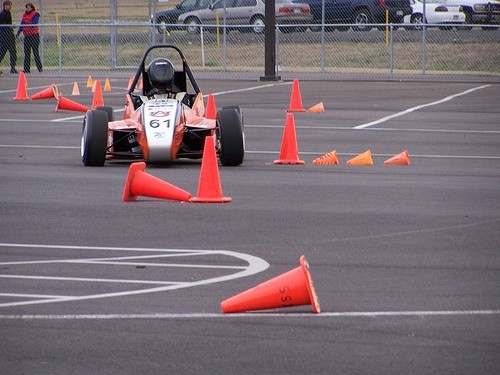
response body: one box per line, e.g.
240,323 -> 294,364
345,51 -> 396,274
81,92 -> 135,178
146,58 -> 181,94
0,0 -> 18,73
16,3 -> 42,72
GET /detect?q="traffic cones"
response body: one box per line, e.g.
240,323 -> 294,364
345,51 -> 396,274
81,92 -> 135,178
91,80 -> 104,109
274,113 -> 304,164
31,83 -> 59,100
87,75 -> 143,92
347,149 -> 373,166
188,135 -> 233,202
55,92 -> 89,112
13,72 -> 31,100
313,150 -> 339,166
384,149 -> 411,164
122,161 -> 191,202
221,255 -> 320,314
287,78 -> 306,112
307,102 -> 325,112
72,81 -> 81,96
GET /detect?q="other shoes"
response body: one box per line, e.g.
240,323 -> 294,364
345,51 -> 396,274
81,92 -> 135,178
0,68 -> 18,73
23,71 -> 31,73
38,67 -> 42,72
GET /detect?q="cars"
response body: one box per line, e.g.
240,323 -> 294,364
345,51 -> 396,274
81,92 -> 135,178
276,0 -> 413,33
418,0 -> 500,32
149,0 -> 217,34
173,0 -> 313,34
395,0 -> 466,31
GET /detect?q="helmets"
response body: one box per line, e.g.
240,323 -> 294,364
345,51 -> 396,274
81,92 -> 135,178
148,59 -> 175,88
